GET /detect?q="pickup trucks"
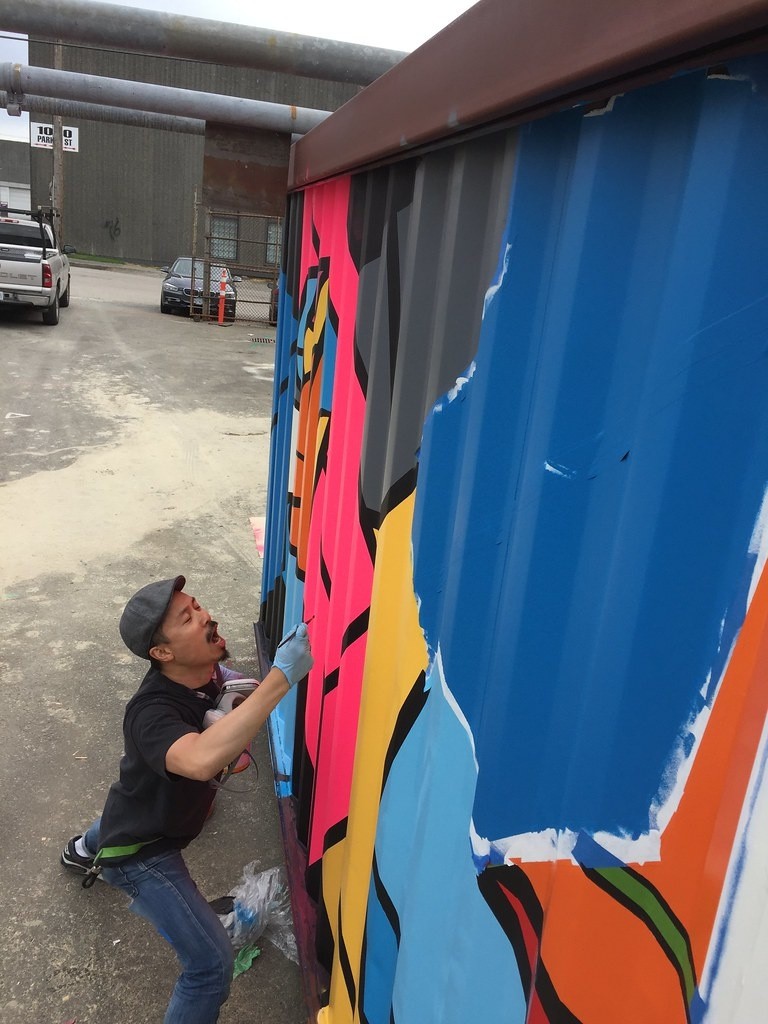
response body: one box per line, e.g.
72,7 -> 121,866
1,216 -> 76,325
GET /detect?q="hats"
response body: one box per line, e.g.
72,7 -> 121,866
119,574 -> 186,660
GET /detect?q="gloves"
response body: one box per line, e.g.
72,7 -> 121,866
271,623 -> 313,689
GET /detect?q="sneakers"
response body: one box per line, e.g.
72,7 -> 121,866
60,835 -> 104,881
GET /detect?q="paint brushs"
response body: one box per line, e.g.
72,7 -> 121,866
278,615 -> 316,650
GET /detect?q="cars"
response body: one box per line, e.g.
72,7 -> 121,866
266,278 -> 281,324
159,256 -> 241,322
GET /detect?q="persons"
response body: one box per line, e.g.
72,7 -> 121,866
56,574 -> 315,1024
